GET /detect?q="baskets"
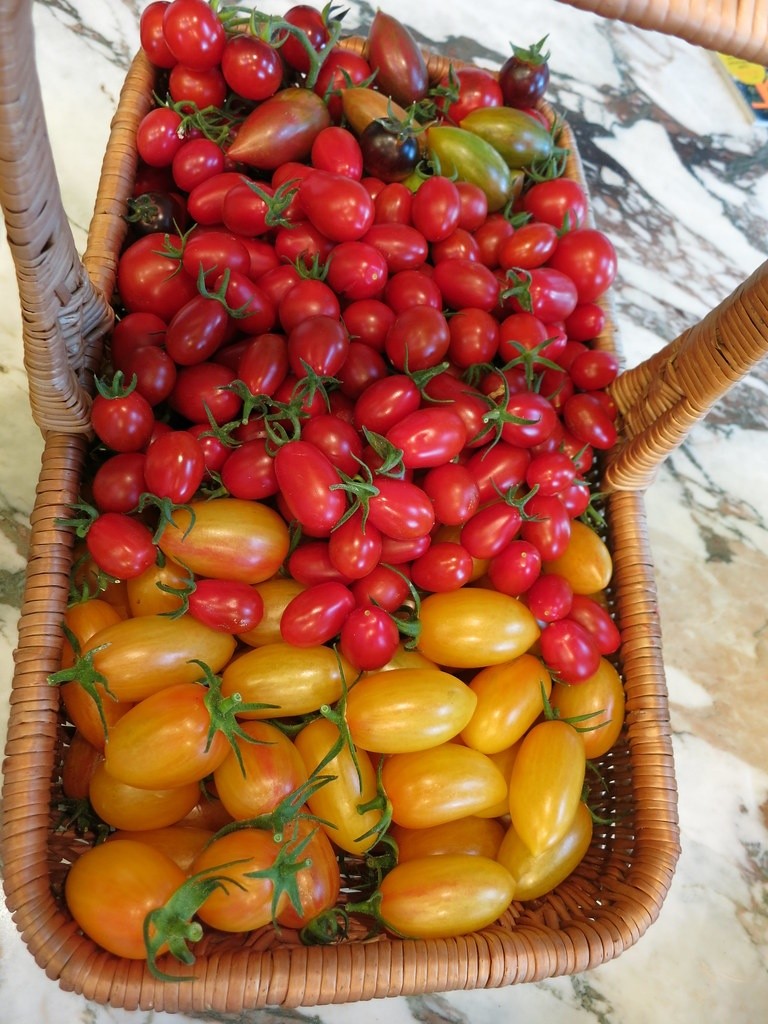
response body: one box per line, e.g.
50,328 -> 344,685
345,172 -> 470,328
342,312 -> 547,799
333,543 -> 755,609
0,0 -> 768,1013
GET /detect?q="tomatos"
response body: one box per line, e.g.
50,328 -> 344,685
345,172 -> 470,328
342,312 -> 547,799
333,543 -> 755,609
58,0 -> 624,960
